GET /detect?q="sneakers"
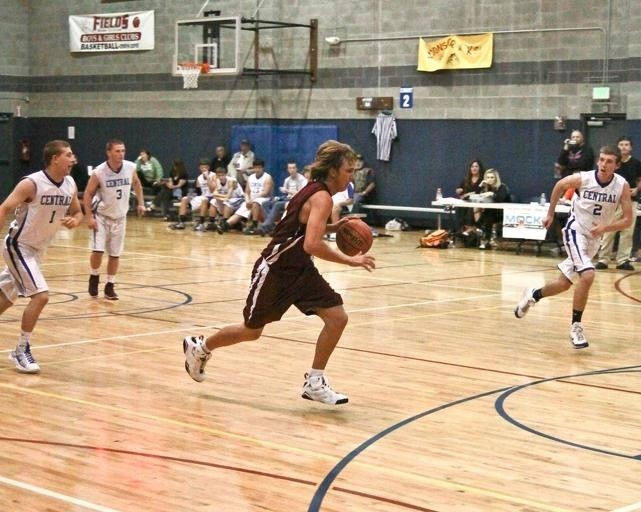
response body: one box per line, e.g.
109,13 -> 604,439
616,262 -> 633,270
104,282 -> 119,300
568,322 -> 588,349
515,287 -> 537,318
170,220 -> 225,234
595,262 -> 608,269
301,372 -> 348,405
463,228 -> 490,249
9,341 -> 40,373
88,274 -> 99,296
243,224 -> 257,234
183,335 -> 212,382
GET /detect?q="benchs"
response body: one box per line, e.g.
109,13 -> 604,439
129,175 -> 220,227
357,196 -> 459,242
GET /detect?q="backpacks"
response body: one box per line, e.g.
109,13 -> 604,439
420,229 -> 452,249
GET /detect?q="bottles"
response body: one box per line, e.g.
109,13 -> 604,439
541,193 -> 547,207
436,189 -> 443,201
554,163 -> 562,179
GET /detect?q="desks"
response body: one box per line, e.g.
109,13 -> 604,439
431,196 -> 591,259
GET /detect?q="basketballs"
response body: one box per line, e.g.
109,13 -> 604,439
336,219 -> 372,255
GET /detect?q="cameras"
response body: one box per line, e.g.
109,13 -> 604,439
567,141 -> 577,144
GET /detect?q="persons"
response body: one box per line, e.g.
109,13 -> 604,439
514,142 -> 635,349
82,138 -> 146,301
554,130 -> 640,271
170,140 -> 375,234
183,139 -> 378,404
2,138 -> 85,374
449,160 -> 510,248
137,150 -> 187,221
